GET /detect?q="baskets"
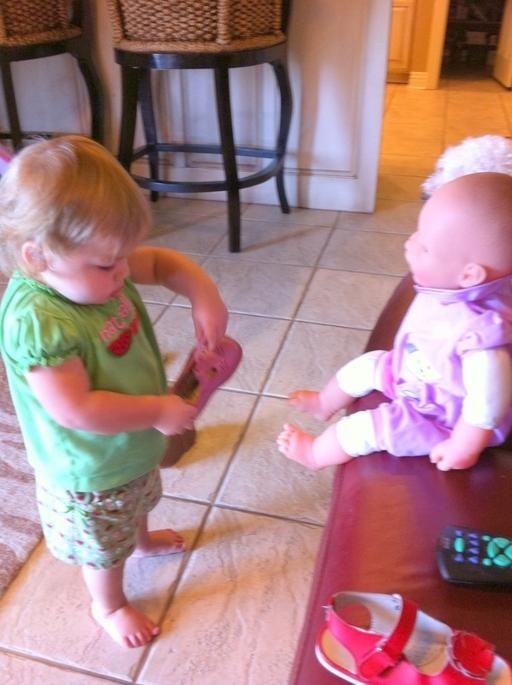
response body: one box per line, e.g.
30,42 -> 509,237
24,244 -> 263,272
107,1 -> 285,52
0,0 -> 82,47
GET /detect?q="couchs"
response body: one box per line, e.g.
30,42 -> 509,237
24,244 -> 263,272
288,267 -> 512,685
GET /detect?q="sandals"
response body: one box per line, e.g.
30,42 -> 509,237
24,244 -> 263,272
314,591 -> 512,685
170,337 -> 243,414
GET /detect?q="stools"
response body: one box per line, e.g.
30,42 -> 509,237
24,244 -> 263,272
0,38 -> 103,153
111,44 -> 294,253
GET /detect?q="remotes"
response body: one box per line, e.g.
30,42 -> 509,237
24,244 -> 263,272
438,523 -> 512,590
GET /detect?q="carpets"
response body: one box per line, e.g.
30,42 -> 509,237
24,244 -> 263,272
1,268 -> 168,602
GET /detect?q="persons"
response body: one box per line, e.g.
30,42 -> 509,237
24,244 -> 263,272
1,136 -> 230,649
275,173 -> 512,472
